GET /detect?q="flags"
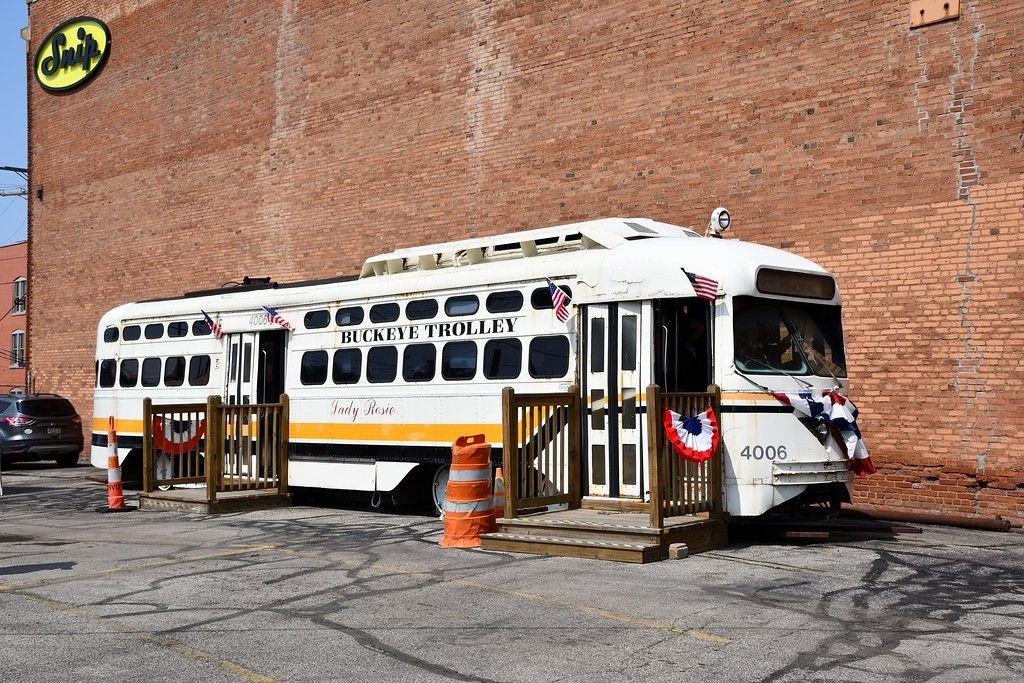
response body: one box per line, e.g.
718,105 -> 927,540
546,277 -> 571,324
201,309 -> 222,340
260,306 -> 289,329
681,267 -> 719,301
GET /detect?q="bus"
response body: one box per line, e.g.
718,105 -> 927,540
90,206 -> 852,518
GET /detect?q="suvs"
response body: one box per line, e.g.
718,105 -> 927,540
0,393 -> 85,471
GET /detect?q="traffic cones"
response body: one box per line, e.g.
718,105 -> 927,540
95,416 -> 138,513
